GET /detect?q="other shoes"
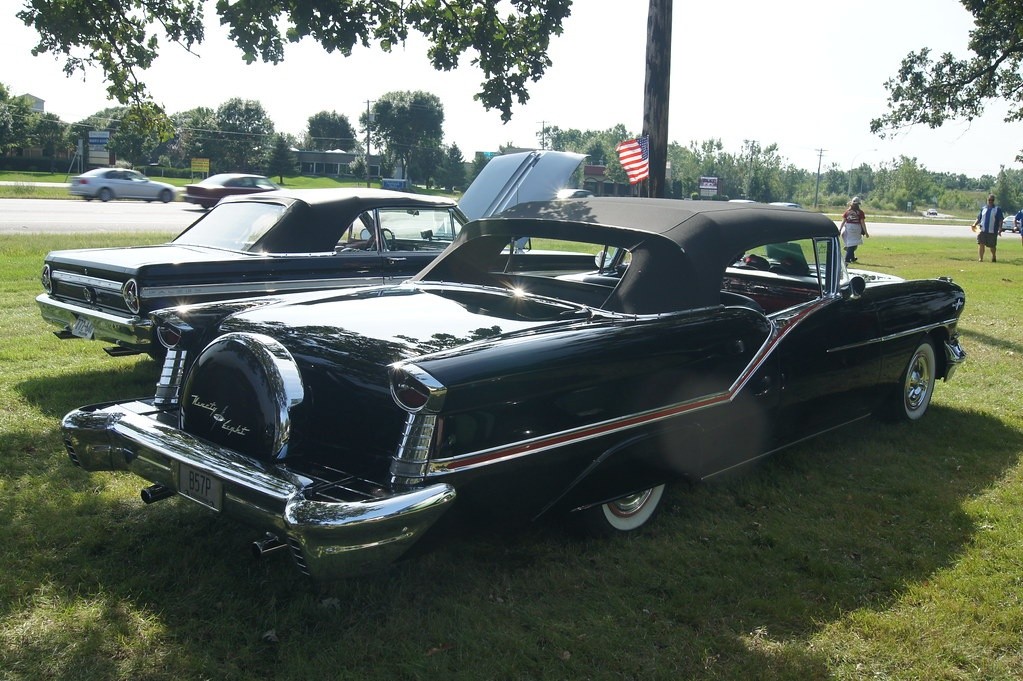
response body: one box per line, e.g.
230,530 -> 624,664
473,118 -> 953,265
844,257 -> 858,267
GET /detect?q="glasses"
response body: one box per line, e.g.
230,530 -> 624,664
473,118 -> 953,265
988,199 -> 995,201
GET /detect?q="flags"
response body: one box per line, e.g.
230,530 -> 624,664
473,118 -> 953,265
615,136 -> 651,185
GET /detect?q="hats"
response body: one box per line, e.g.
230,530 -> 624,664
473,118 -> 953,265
847,197 -> 861,205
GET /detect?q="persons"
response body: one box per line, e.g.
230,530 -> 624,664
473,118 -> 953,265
1013,207 -> 1023,248
837,198 -> 869,267
972,194 -> 1004,262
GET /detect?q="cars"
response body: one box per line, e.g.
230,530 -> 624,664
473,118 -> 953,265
1001,216 -> 1021,233
770,202 -> 801,208
34,151 -> 590,359
728,200 -> 756,204
62,198 -> 967,582
68,168 -> 178,203
180,174 -> 288,209
927,209 -> 937,216
